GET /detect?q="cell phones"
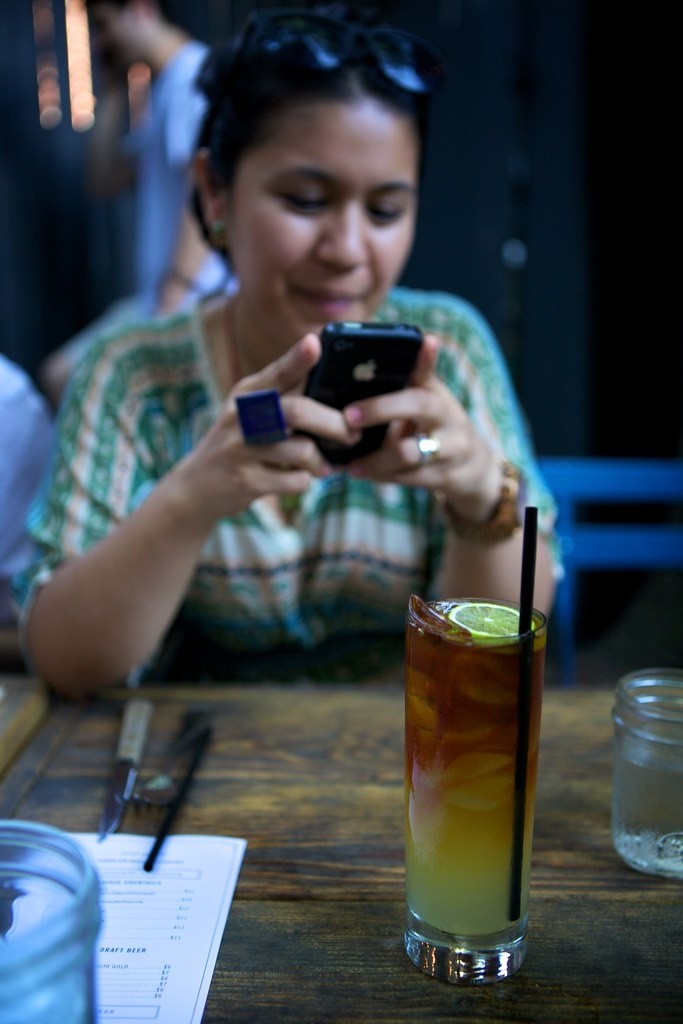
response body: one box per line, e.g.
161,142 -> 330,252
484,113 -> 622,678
293,321 -> 425,467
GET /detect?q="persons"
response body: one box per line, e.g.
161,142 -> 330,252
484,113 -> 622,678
0,0 -> 242,676
0,8 -> 560,695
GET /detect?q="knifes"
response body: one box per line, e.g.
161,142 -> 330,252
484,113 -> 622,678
98,698 -> 153,840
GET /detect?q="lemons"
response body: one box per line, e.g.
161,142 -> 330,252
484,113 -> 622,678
447,601 -> 536,646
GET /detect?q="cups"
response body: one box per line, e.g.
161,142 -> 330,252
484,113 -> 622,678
404,598 -> 548,989
611,666 -> 683,880
1,818 -> 102,1024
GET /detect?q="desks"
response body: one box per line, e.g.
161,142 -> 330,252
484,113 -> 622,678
0,672 -> 683,1024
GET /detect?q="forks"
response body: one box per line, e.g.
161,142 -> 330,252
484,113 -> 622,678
113,711 -> 203,806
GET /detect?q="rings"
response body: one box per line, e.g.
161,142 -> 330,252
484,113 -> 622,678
414,431 -> 439,464
237,390 -> 296,458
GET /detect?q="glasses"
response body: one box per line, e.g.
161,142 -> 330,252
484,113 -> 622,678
202,13 -> 435,131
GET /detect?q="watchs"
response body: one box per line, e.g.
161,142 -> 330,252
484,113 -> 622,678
434,453 -> 531,540
